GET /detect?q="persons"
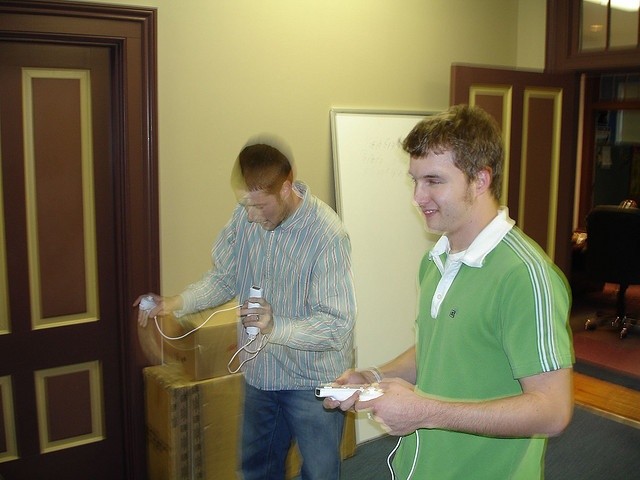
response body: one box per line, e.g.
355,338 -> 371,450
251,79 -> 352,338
130,143 -> 359,479
316,106 -> 577,479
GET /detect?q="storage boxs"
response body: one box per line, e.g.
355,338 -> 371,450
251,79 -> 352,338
142,365 -> 357,480
163,301 -> 239,380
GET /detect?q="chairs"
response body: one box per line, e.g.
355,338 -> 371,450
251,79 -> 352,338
584,206 -> 640,340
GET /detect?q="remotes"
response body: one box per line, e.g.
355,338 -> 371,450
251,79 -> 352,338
246,284 -> 263,335
314,387 -> 384,401
